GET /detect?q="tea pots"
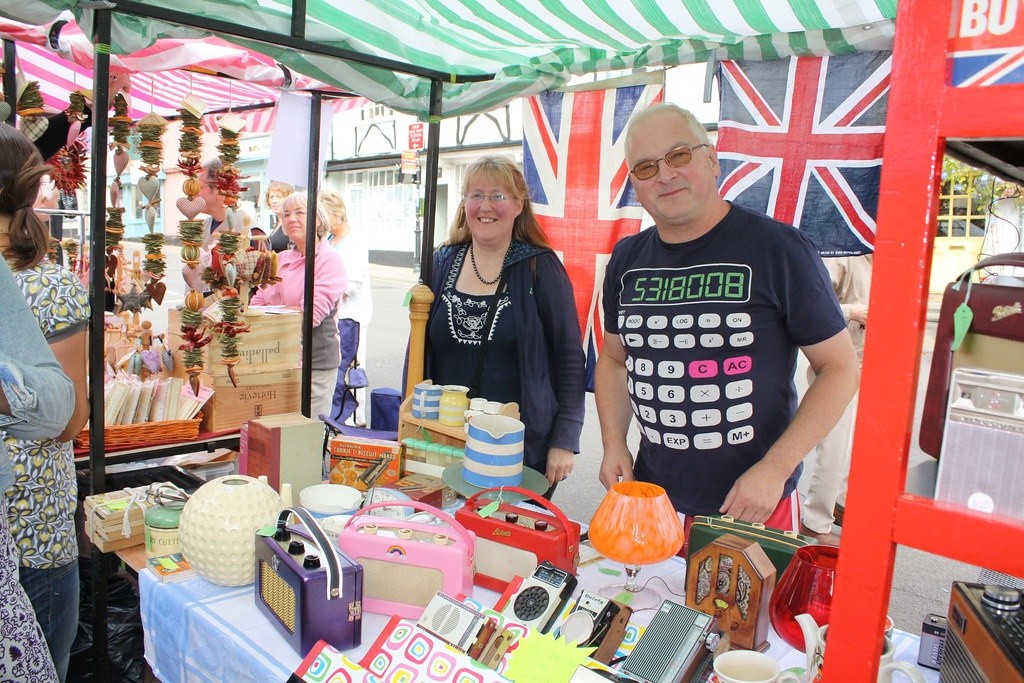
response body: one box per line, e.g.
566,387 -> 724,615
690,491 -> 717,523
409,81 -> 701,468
796,611 -> 926,683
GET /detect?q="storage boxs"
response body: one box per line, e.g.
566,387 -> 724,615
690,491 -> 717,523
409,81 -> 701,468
167,309 -> 302,433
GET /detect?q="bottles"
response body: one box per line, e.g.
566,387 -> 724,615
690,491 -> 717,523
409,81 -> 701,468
438,386 -> 469,426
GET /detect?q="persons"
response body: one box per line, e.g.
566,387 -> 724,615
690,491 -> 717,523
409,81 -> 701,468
195,157 -> 240,251
0,119 -> 90,683
591,102 -> 863,558
263,179 -> 296,253
0,253 -> 75,683
401,152 -> 585,510
803,250 -> 874,546
314,191 -> 371,426
249,189 -> 347,425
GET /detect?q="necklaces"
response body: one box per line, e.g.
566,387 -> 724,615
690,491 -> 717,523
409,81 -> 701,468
468,242 -> 512,284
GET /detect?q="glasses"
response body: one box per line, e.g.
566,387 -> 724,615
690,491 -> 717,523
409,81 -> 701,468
630,144 -> 709,181
464,191 -> 514,203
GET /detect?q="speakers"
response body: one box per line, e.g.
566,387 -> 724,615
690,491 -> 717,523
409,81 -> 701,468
918,278 -> 1024,524
253,484 -> 819,683
938,578 -> 1024,683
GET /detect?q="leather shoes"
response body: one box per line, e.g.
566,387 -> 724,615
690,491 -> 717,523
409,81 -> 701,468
832,504 -> 844,527
800,518 -> 841,547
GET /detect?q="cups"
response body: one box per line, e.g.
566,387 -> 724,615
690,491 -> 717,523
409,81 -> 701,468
713,648 -> 800,683
462,415 -> 525,488
884,616 -> 894,640
412,383 -> 441,420
300,483 -> 363,523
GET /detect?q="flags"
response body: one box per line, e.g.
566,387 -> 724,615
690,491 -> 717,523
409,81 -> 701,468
521,67 -> 666,394
711,50 -> 893,256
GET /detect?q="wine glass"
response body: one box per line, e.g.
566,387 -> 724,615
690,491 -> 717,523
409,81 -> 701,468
769,545 -> 843,682
588,481 -> 685,611
